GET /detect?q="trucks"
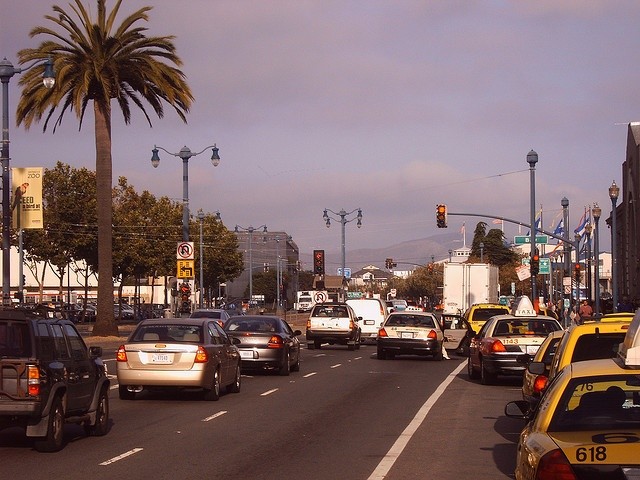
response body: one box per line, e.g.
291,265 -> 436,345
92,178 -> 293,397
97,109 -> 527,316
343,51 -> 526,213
296,290 -> 329,313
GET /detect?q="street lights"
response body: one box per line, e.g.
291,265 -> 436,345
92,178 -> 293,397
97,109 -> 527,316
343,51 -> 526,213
554,253 -> 558,308
0,53 -> 57,308
584,222 -> 592,304
574,231 -> 582,300
233,223 -> 268,308
592,202 -> 602,319
608,179 -> 620,312
151,142 -> 221,241
560,196 -> 569,277
323,207 -> 363,286
526,148 -> 538,314
190,208 -> 222,309
559,250 -> 563,325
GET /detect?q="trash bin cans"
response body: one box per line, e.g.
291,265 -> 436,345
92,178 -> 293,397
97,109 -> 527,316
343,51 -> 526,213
163,309 -> 173,318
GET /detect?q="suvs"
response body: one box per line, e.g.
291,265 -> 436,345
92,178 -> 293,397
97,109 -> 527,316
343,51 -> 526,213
0,308 -> 111,453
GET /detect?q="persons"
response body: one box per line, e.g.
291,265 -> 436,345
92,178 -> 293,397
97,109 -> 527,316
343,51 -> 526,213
580,300 -> 593,319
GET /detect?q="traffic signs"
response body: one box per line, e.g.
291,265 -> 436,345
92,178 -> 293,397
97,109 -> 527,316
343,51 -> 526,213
522,258 -> 550,275
347,292 -> 362,299
337,268 -> 351,279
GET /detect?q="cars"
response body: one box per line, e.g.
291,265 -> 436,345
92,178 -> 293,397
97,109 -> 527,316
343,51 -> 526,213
504,306 -> 640,480
516,330 -> 565,405
375,306 -> 447,359
223,315 -> 302,376
116,318 -> 242,401
467,295 -> 565,384
189,308 -> 230,322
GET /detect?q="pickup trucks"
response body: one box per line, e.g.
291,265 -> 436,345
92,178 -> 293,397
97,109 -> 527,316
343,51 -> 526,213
306,302 -> 363,351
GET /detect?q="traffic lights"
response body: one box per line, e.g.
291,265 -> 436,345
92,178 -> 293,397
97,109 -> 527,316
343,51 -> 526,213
315,253 -> 323,271
436,207 -> 439,227
533,256 -> 538,274
574,263 -> 582,271
437,205 -> 445,227
429,265 -> 432,274
385,260 -> 387,268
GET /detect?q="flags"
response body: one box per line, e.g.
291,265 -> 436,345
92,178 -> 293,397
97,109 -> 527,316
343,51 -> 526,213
492,217 -> 503,225
549,218 -> 564,238
459,221 -> 466,235
525,218 -> 541,235
574,217 -> 590,237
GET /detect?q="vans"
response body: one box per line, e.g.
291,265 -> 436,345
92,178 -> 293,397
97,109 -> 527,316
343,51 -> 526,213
528,312 -> 635,404
389,299 -> 407,310
462,302 -> 511,334
344,297 -> 388,343
